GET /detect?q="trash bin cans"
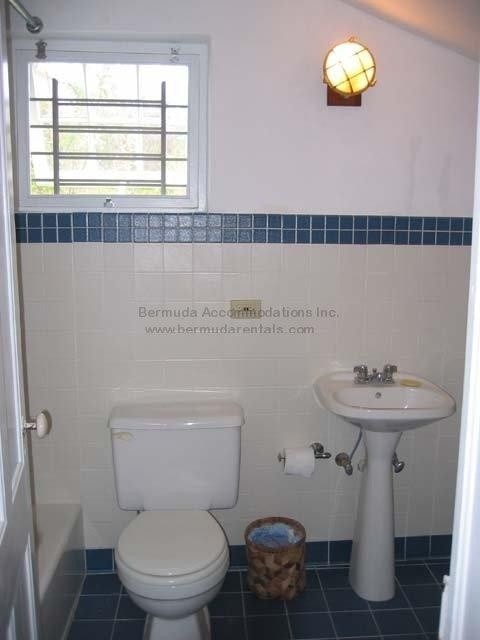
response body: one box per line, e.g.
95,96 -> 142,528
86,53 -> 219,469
245,516 -> 306,602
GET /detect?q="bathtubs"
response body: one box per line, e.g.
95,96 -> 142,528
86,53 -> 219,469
32,503 -> 87,639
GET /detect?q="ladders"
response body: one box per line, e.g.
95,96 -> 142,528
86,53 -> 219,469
52,78 -> 167,196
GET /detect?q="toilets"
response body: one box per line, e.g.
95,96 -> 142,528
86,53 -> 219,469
107,402 -> 245,640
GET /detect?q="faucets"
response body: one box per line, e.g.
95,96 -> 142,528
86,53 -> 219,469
371,368 -> 383,384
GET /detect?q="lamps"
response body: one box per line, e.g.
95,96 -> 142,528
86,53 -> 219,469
322,36 -> 377,106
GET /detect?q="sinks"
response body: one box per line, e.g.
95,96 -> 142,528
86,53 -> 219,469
313,370 -> 456,431
